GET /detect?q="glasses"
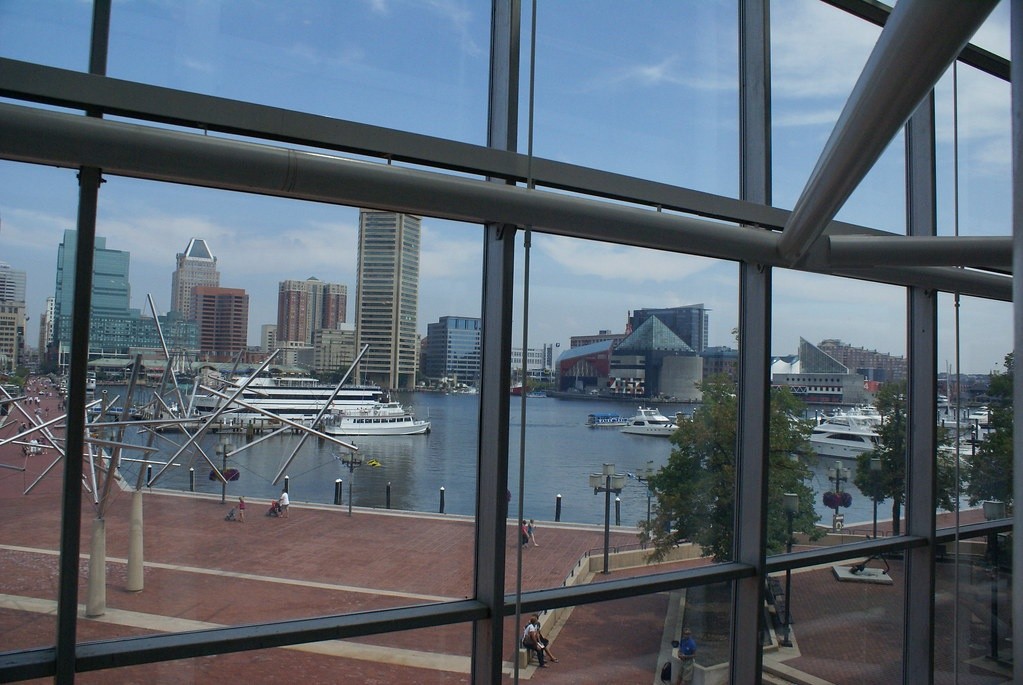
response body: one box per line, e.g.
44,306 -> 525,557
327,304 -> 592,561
685,632 -> 691,635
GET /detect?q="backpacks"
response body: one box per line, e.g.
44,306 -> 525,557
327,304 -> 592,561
660,662 -> 671,684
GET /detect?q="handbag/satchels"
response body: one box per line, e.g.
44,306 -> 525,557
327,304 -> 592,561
541,638 -> 549,647
523,627 -> 537,649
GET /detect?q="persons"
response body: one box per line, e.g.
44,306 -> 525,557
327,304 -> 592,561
522,613 -> 559,668
522,518 -> 540,549
90,434 -> 97,463
23,375 -> 66,417
672,627 -> 698,685
271,489 -> 290,518
18,420 -> 48,457
232,496 -> 246,523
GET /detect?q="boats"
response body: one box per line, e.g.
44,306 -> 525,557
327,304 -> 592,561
937,395 -> 993,455
324,402 -> 431,436
186,376 -> 405,423
668,412 -> 693,424
510,382 -> 529,395
620,406 -> 679,437
796,404 -> 892,461
585,413 -> 628,427
526,391 -> 547,398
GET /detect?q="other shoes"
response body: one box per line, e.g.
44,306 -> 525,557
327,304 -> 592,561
542,664 -> 549,667
553,658 -> 559,663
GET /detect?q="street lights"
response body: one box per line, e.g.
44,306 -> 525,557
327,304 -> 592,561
342,441 -> 365,517
781,493 -> 799,647
635,461 -> 664,537
590,464 -> 626,574
215,438 -> 236,504
828,461 -> 850,514
983,501 -> 1006,659
